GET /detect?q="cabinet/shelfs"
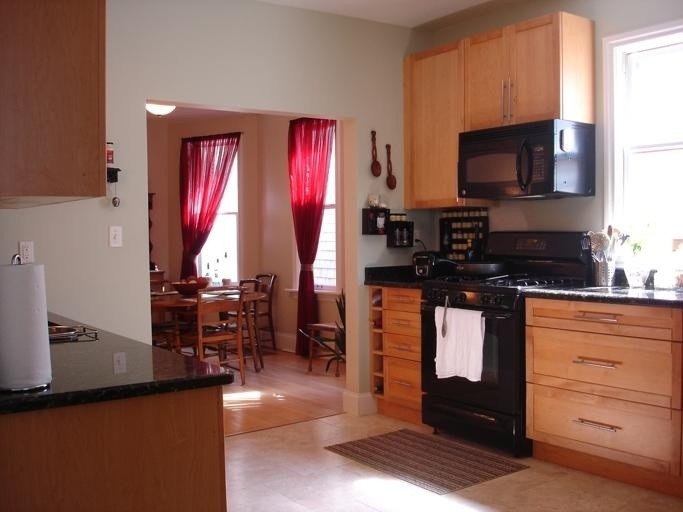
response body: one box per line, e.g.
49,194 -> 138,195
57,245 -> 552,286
402,39 -> 498,210
0,385 -> 230,512
0,0 -> 107,213
524,297 -> 683,499
368,285 -> 423,428
462,10 -> 597,132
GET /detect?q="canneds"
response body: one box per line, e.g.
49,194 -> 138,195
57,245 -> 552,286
390,214 -> 407,222
442,208 -> 488,262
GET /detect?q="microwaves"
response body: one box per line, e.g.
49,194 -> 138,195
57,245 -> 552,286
458,119 -> 595,201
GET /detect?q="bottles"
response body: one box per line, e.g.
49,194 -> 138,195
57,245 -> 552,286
222,278 -> 230,286
370,212 -> 411,247
161,284 -> 166,292
155,264 -> 158,271
610,258 -> 629,288
440,207 -> 489,264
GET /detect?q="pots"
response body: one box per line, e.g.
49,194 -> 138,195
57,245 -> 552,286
434,257 -> 504,280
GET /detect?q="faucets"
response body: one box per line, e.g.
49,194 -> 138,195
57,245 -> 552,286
645,268 -> 659,288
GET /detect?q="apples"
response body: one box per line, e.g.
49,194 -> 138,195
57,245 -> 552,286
172,276 -> 209,285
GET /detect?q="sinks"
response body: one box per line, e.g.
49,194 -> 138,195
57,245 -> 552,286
576,284 -> 680,301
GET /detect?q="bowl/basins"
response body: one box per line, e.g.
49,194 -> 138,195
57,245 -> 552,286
170,283 -> 208,296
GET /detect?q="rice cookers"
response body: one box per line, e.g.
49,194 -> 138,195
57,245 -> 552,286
412,252 -> 439,279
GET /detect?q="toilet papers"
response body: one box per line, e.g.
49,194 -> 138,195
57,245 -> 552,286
0,262 -> 53,393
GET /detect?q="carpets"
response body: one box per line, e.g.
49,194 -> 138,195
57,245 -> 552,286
323,429 -> 531,496
218,378 -> 347,437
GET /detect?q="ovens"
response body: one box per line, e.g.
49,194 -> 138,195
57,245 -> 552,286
420,302 -> 526,458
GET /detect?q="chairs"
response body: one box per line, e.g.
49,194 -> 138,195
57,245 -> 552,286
150,270 -> 276,385
306,321 -> 344,378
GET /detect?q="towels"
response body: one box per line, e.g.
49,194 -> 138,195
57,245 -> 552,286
434,306 -> 485,382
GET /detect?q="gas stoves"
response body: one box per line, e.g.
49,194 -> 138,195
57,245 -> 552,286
421,271 -> 589,312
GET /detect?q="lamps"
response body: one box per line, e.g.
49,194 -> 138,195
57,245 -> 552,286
145,103 -> 176,119
